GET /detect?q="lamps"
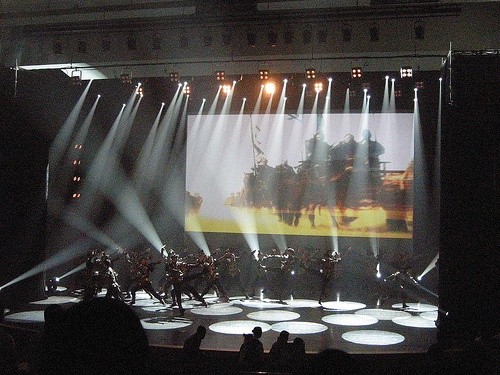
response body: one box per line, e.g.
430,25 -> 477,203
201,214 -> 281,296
45,276 -> 60,296
49,19 -> 426,56
71,65 -> 421,100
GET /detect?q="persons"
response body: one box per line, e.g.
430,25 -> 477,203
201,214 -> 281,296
187,129 -> 391,216
171,322 -> 500,374
80,241 -> 423,312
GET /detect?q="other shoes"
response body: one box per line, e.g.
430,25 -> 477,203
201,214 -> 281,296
77,284 -> 418,316
